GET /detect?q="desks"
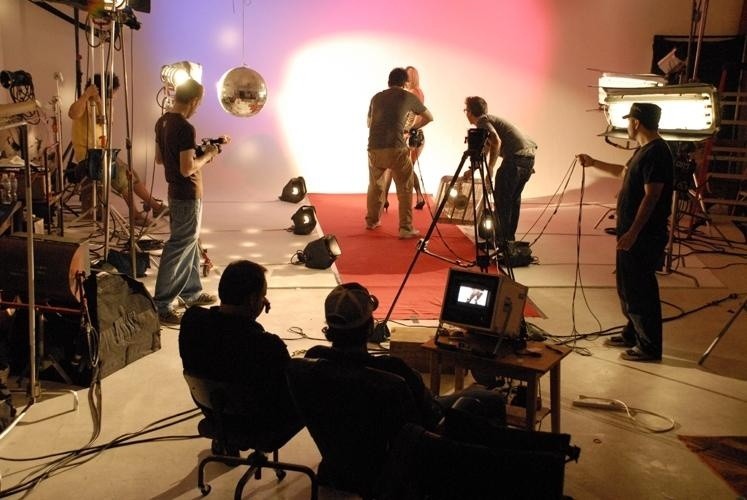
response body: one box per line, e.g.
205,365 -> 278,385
421,339 -> 573,433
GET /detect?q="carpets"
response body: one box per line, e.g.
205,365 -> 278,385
677,436 -> 747,500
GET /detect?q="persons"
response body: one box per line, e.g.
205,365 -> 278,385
287,282 -> 505,500
365,68 -> 431,239
178,260 -> 290,453
463,95 -> 537,241
575,101 -> 672,362
68,72 -> 169,228
153,78 -> 231,325
382,66 -> 426,210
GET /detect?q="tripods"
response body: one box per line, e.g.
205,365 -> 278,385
66,18 -> 129,226
383,154 -> 516,326
1,123 -> 81,441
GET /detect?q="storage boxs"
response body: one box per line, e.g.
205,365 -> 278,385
3,169 -> 51,199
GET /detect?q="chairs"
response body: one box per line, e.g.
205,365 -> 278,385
183,369 -> 318,500
287,357 -> 418,500
401,423 -> 571,500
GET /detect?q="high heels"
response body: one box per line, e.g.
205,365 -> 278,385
412,200 -> 426,210
383,201 -> 389,212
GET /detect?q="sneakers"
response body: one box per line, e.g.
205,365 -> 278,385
157,308 -> 185,325
398,229 -> 420,239
178,293 -> 218,306
618,345 -> 661,364
603,335 -> 634,347
365,220 -> 383,230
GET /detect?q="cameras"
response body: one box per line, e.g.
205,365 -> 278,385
464,127 -> 486,150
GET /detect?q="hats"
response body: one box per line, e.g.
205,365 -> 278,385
323,281 -> 379,330
620,102 -> 663,130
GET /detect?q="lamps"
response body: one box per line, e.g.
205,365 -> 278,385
291,205 -> 317,235
290,234 -> 342,270
156,60 -> 204,118
478,208 -> 496,239
587,67 -> 667,108
444,184 -> 468,210
587,81 -> 719,141
278,176 -> 307,203
